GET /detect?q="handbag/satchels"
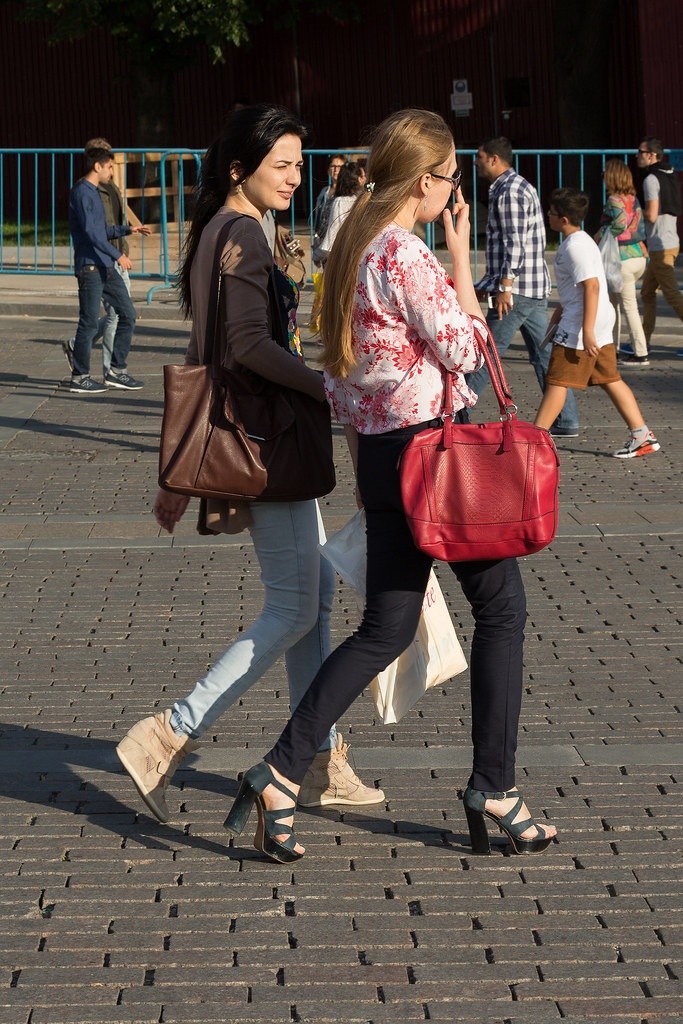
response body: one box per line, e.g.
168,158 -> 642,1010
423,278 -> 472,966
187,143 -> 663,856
309,272 -> 323,332
158,363 -> 337,501
360,523 -> 469,724
398,314 -> 560,561
598,225 -> 623,293
321,509 -> 364,602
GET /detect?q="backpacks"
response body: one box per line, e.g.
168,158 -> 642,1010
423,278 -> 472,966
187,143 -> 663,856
274,224 -> 306,289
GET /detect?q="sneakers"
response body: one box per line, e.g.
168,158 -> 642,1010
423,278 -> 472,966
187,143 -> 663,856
613,422 -> 661,459
62,339 -> 74,372
105,369 -> 145,390
70,376 -> 107,392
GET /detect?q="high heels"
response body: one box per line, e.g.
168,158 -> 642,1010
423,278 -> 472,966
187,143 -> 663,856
223,760 -> 304,864
463,787 -> 554,855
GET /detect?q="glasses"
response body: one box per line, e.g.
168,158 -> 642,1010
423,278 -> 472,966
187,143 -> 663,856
639,149 -> 652,154
429,167 -> 463,191
328,165 -> 342,170
548,210 -> 562,217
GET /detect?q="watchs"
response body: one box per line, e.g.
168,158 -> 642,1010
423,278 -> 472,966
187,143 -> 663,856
499,284 -> 512,292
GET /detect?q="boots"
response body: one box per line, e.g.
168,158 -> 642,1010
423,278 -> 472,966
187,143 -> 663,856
297,733 -> 385,806
114,707 -> 200,824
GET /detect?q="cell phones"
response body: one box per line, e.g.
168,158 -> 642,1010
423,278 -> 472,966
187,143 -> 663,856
488,294 -> 514,308
539,324 -> 558,351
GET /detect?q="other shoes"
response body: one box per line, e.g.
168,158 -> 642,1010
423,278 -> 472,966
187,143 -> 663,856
620,355 -> 650,365
619,343 -> 651,354
550,425 -> 577,438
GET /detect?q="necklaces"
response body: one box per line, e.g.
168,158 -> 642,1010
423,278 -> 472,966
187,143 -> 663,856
394,221 -> 403,228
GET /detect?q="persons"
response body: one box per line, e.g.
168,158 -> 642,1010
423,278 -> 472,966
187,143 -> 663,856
61,139 -> 130,385
620,139 -> 683,357
224,108 -> 559,865
466,137 -> 579,438
114,105 -> 384,824
67,149 -> 152,393
594,160 -> 650,366
311,156 -> 365,345
534,188 -> 660,459
